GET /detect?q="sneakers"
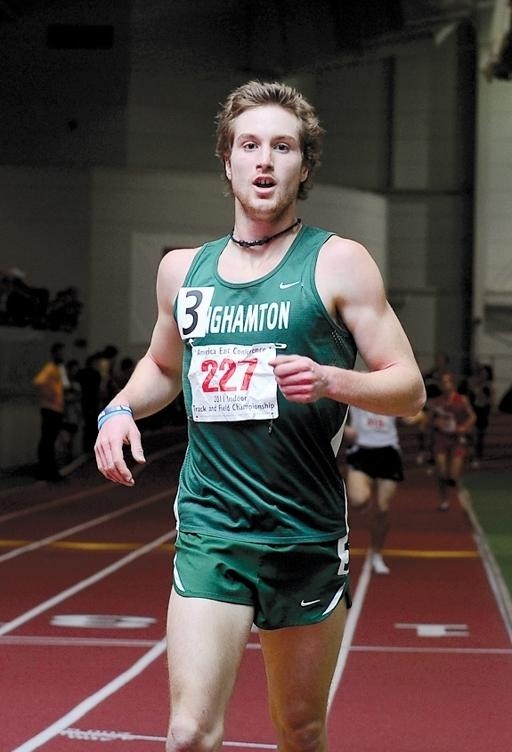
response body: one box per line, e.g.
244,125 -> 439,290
370,553 -> 389,577
440,499 -> 450,510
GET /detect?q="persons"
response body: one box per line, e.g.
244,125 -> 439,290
93,81 -> 429,752
344,359 -> 495,576
32,341 -> 184,482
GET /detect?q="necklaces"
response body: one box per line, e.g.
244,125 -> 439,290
229,219 -> 303,247
